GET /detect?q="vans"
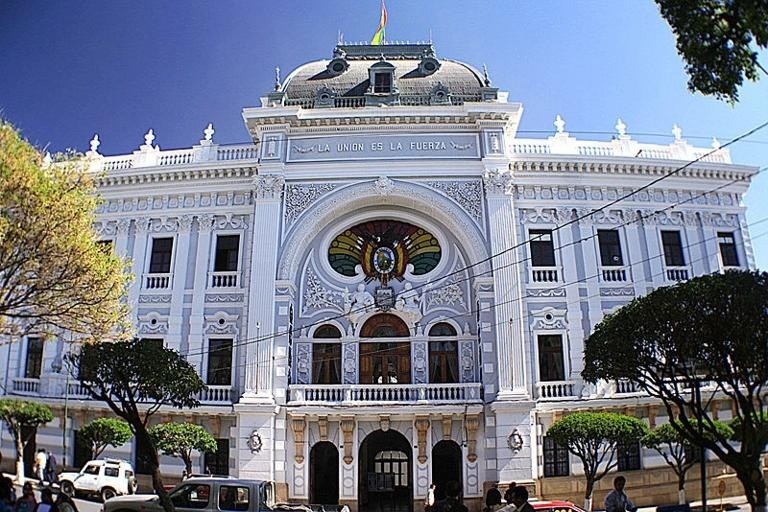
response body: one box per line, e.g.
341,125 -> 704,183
182,473 -> 244,499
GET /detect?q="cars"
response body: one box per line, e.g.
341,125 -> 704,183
492,500 -> 586,512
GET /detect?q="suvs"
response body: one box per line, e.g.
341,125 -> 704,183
59,457 -> 138,502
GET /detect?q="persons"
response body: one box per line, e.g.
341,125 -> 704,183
396,281 -> 423,314
420,478 -> 535,511
601,473 -> 637,511
349,282 -> 376,320
1,446 -> 78,511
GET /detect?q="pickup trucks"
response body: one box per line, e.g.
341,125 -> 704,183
101,477 -> 350,512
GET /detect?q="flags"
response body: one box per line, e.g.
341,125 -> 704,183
370,10 -> 387,45
